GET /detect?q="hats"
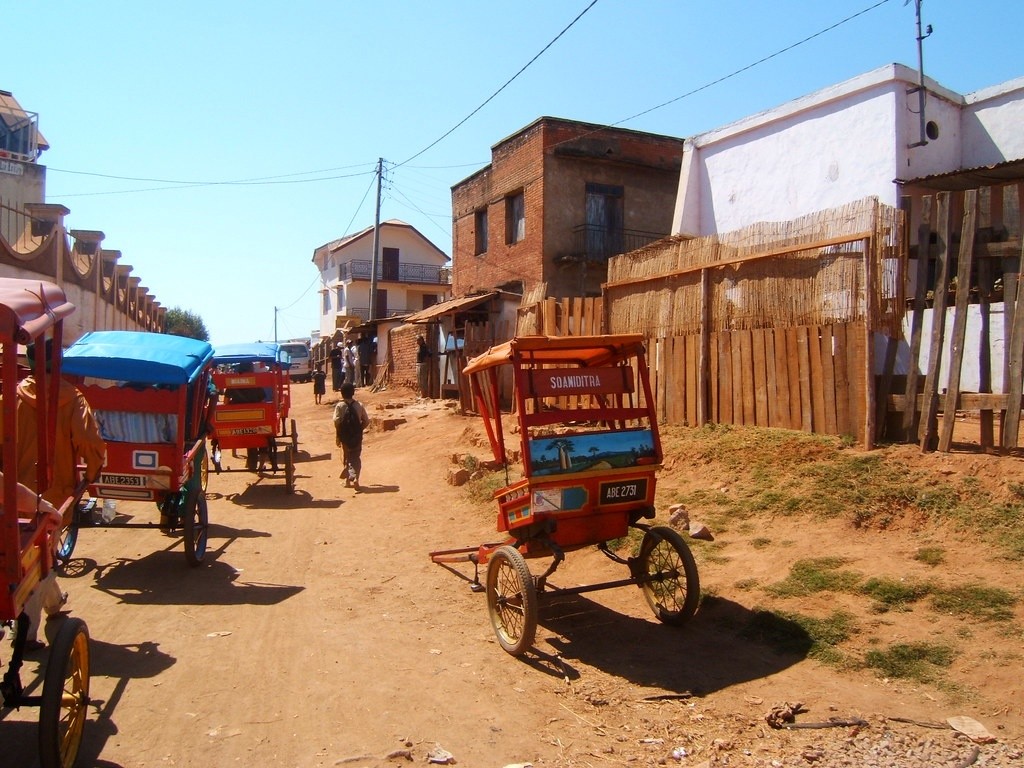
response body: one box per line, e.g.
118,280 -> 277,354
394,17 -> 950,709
337,342 -> 344,347
346,340 -> 353,343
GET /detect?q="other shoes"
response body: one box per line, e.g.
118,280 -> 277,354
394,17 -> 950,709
354,480 -> 359,490
340,481 -> 351,488
47,591 -> 68,615
10,639 -> 44,649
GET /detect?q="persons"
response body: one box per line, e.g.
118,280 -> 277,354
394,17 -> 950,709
358,335 -> 374,387
311,361 -> 326,405
94,381 -> 177,444
343,339 -> 355,385
0,336 -> 105,526
224,363 -> 265,404
330,342 -> 344,390
0,471 -> 63,531
351,339 -> 361,388
158,372 -> 219,452
416,335 -> 432,398
334,382 -> 369,491
247,361 -> 275,470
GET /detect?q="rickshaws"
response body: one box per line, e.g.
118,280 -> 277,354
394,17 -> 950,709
202,340 -> 300,493
426,333 -> 701,654
45,330 -> 218,565
0,275 -> 112,768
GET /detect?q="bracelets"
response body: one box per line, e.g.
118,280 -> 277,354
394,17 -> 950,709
37,493 -> 43,514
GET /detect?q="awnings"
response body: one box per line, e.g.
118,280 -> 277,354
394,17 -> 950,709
403,293 -> 495,322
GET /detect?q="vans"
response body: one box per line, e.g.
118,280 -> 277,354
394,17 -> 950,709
279,342 -> 313,381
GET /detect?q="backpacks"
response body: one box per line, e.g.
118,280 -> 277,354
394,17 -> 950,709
340,400 -> 361,446
